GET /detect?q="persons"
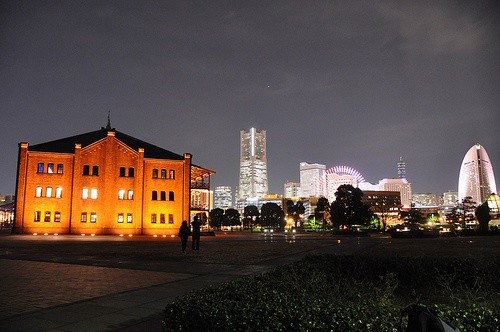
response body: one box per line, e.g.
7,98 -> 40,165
189,215 -> 204,250
178,220 -> 191,253
